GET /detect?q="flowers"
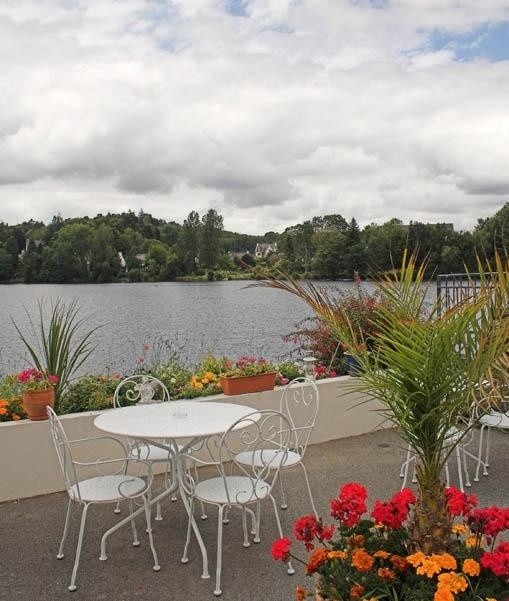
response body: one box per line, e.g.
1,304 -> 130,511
60,367 -> 221,412
12,364 -> 60,392
270,482 -> 509,600
278,366 -> 337,385
213,355 -> 276,378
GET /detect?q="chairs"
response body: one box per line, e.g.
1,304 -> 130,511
112,373 -> 207,521
46,406 -> 160,592
175,409 -> 295,597
233,376 -> 321,544
396,375 -> 509,496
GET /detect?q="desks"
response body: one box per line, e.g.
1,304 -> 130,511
93,400 -> 260,579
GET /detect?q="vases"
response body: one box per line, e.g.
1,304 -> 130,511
18,385 -> 59,425
219,371 -> 276,396
